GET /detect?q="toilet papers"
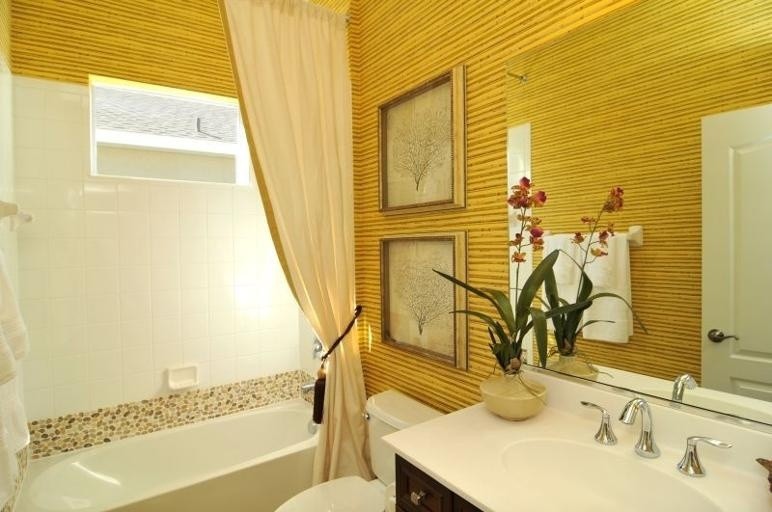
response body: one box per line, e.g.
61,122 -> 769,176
383,484 -> 395,512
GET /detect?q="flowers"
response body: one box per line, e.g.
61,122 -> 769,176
541,188 -> 649,348
428,175 -> 591,370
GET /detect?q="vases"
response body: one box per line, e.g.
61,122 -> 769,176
533,350 -> 600,376
479,368 -> 550,421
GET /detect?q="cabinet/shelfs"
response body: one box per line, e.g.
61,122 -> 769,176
504,0 -> 771,422
396,454 -> 479,512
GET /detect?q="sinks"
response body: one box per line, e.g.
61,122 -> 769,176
500,439 -> 724,512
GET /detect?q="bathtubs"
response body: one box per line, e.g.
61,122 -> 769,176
13,398 -> 329,512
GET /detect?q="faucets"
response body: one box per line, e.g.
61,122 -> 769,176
639,390 -> 772,432
618,397 -> 661,459
301,383 -> 316,393
671,374 -> 698,409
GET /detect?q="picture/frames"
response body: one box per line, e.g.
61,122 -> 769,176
376,67 -> 466,216
375,228 -> 472,369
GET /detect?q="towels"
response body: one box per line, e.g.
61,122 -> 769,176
539,235 -> 639,344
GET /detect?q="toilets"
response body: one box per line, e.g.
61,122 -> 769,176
275,389 -> 445,512
